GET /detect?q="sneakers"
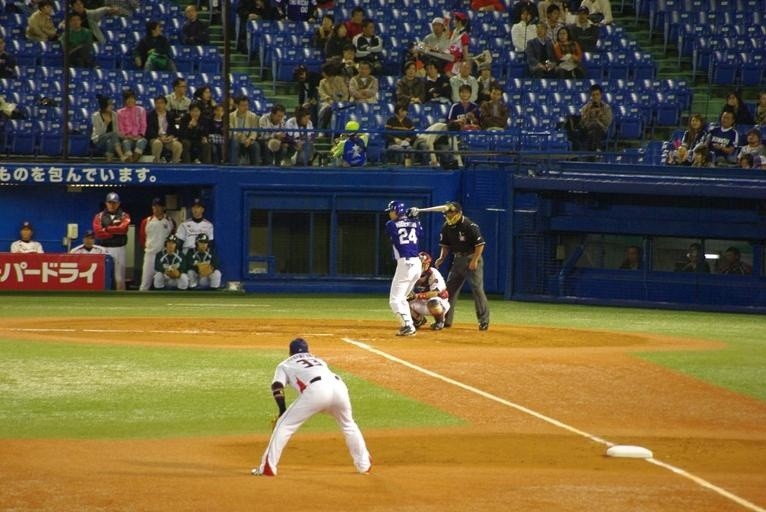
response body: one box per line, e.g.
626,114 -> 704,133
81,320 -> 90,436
414,317 -> 426,328
479,322 -> 488,330
396,326 -> 416,336
431,320 -> 450,329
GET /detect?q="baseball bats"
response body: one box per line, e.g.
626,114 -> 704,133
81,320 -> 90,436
407,205 -> 448,213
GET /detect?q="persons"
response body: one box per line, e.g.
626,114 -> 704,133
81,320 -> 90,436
470,0 -> 613,78
250,338 -> 372,476
239,0 -> 336,22
178,6 -> 210,46
582,84 -> 613,151
665,86 -> 766,169
435,201 -> 489,331
91,76 -> 315,170
385,10 -> 509,169
26,0 -> 133,71
290,6 -> 384,138
92,192 -> 132,290
131,18 -> 177,73
10,221 -> 44,254
139,198 -> 175,290
384,198 -> 423,336
328,121 -> 371,168
68,233 -> 105,255
406,251 -> 450,330
186,233 -> 222,290
153,234 -> 189,291
174,198 -> 214,256
1,37 -> 28,120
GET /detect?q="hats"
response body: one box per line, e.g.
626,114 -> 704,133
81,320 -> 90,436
153,198 -> 163,206
433,17 -> 445,24
19,221 -> 32,230
290,338 -> 308,353
106,192 -> 119,203
85,230 -> 95,239
191,198 -> 204,207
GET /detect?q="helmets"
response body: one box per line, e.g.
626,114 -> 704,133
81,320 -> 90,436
385,200 -> 409,215
195,234 -> 209,249
346,122 -> 361,134
443,201 -> 463,226
418,251 -> 432,271
164,234 -> 179,248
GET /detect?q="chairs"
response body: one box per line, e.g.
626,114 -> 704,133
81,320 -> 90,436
0,0 -> 766,165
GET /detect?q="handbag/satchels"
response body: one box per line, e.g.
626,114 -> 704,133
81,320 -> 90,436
149,53 -> 167,67
